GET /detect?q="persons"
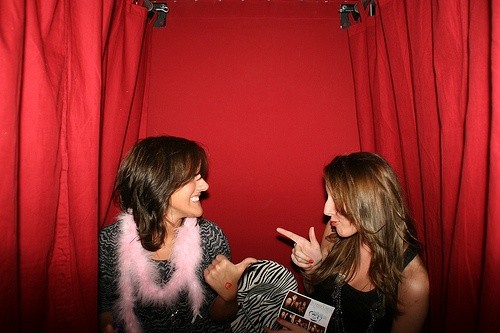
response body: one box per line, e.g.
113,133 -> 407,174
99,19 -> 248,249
98,136 -> 258,333
262,152 -> 429,333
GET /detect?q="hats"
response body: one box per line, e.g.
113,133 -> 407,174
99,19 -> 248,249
231,259 -> 298,332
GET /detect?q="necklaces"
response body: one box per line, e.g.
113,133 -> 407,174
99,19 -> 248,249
333,270 -> 385,333
